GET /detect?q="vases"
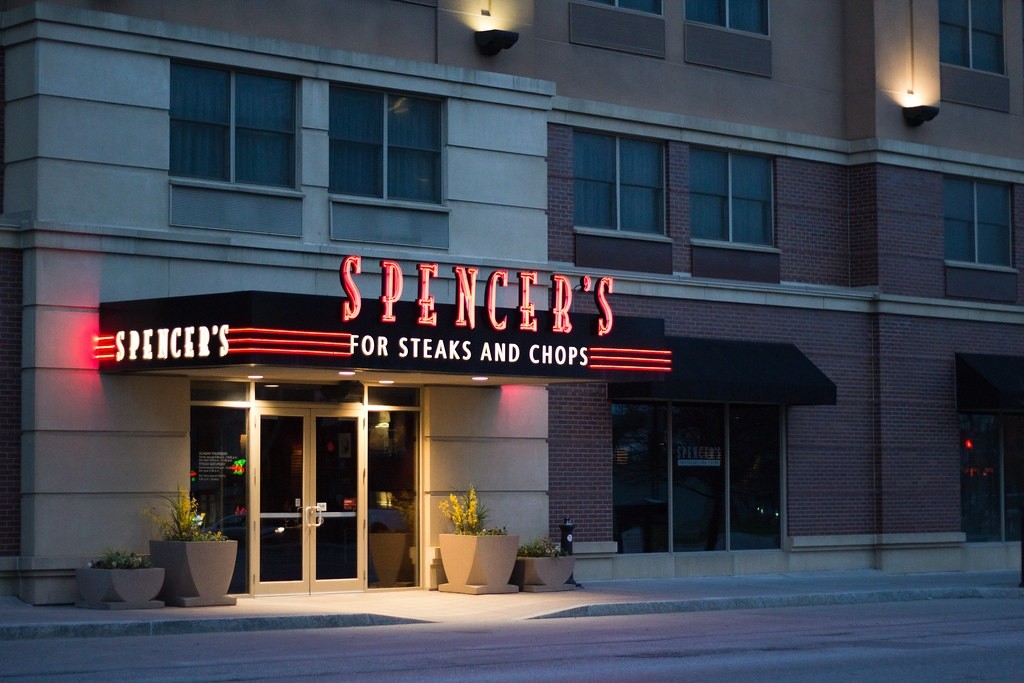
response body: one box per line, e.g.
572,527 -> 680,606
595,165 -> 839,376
149,539 -> 239,607
439,534 -> 521,595
514,556 -> 577,593
140,483 -> 222,540
76,567 -> 165,609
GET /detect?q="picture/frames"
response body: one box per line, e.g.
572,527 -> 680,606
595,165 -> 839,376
338,433 -> 352,457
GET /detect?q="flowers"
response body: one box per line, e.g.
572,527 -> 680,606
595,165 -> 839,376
88,546 -> 152,569
520,538 -> 560,557
439,483 -> 490,535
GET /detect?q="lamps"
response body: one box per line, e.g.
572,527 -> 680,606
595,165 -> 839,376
474,30 -> 519,56
902,106 -> 940,127
